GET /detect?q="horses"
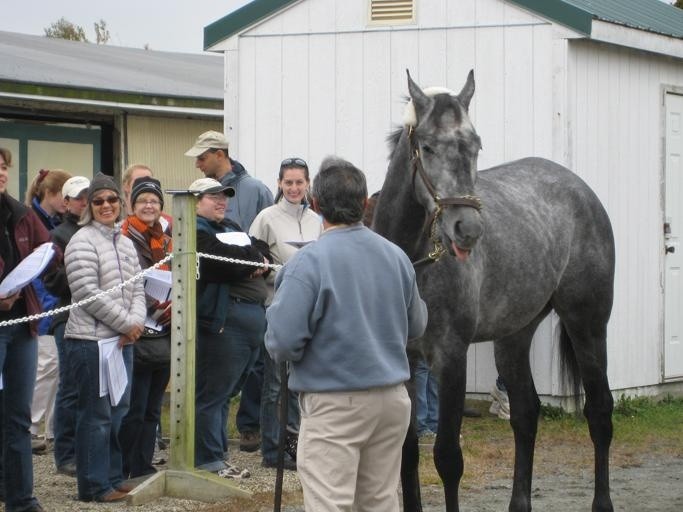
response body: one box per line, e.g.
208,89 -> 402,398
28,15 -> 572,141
363,67 -> 615,512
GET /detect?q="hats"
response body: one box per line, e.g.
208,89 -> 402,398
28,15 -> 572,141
61,176 -> 92,200
86,172 -> 120,201
130,181 -> 164,211
188,178 -> 235,199
184,130 -> 230,159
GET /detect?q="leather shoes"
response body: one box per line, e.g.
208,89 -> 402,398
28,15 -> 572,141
56,463 -> 78,477
92,487 -> 126,502
115,482 -> 137,493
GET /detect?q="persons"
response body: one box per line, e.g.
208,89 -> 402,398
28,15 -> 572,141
248,156 -> 323,471
489,375 -> 510,422
415,359 -> 463,442
27,165 -> 173,503
184,128 -> 274,453
264,154 -> 429,512
0,147 -> 60,511
186,177 -> 271,478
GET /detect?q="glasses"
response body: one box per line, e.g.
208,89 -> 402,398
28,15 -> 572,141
90,196 -> 119,206
133,197 -> 162,206
279,158 -> 307,168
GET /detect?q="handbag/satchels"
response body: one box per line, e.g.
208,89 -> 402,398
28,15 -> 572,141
135,321 -> 171,368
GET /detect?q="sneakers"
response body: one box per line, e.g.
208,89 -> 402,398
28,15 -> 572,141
489,399 -> 513,420
212,460 -> 252,481
417,429 -> 438,447
491,383 -> 514,414
237,430 -> 263,453
259,449 -> 297,472
